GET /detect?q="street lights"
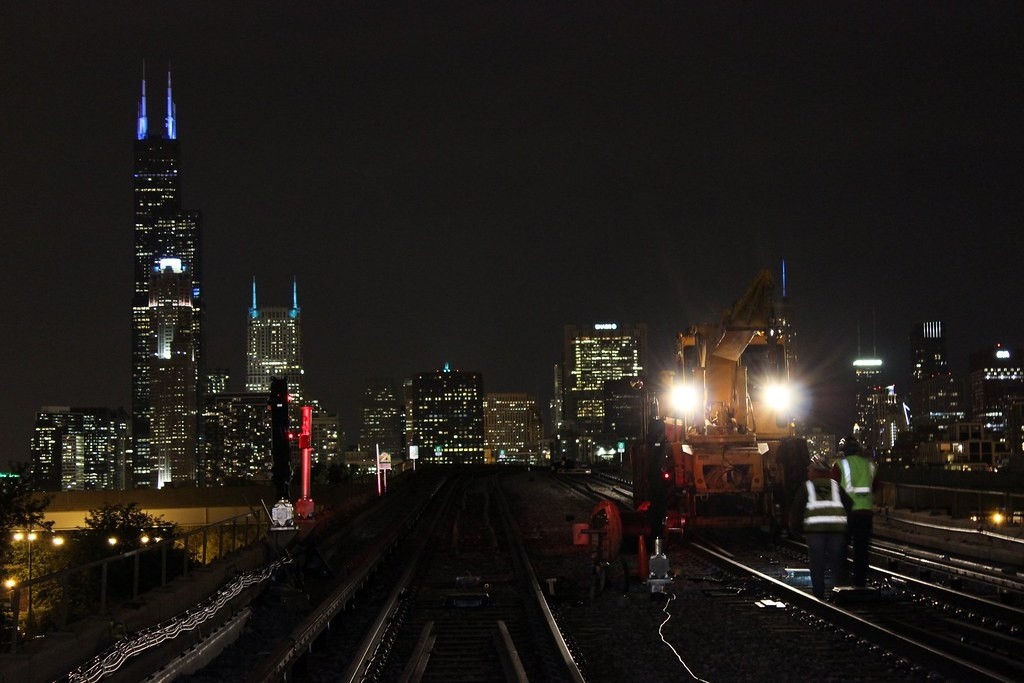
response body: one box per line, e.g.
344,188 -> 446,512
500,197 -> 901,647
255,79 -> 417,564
4,532 -> 164,589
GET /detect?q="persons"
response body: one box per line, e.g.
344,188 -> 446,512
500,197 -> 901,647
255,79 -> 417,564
788,461 -> 854,595
832,435 -> 880,587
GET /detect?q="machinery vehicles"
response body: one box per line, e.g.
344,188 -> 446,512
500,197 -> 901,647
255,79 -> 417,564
633,265 -> 811,545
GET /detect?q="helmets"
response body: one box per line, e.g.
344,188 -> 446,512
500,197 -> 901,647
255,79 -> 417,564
806,458 -> 833,479
837,434 -> 859,452
